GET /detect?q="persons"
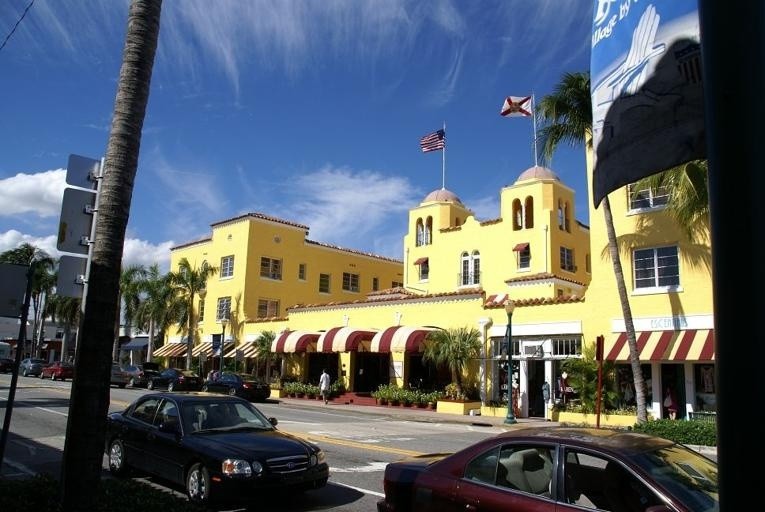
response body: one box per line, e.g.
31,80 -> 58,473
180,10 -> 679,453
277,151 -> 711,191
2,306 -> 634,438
665,385 -> 679,420
318,368 -> 330,405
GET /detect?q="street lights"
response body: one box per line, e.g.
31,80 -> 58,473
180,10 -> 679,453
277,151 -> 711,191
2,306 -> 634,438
216,316 -> 229,372
501,298 -> 518,425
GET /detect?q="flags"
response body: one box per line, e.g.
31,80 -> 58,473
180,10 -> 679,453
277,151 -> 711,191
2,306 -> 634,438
498,94 -> 533,119
419,128 -> 445,154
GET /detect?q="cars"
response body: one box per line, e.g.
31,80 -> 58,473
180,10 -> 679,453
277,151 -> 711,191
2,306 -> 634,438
105,390 -> 331,507
0,356 -> 272,403
377,424 -> 722,512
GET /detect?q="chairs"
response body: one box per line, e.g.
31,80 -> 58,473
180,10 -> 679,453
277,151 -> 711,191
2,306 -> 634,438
198,405 -> 220,428
474,449 -> 554,498
136,405 -> 177,428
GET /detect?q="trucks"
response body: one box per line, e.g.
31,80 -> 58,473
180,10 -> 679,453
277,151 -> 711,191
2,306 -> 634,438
0,341 -> 10,372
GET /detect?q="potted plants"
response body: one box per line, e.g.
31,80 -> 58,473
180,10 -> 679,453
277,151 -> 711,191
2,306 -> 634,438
282,378 -> 346,401
370,384 -> 444,411
